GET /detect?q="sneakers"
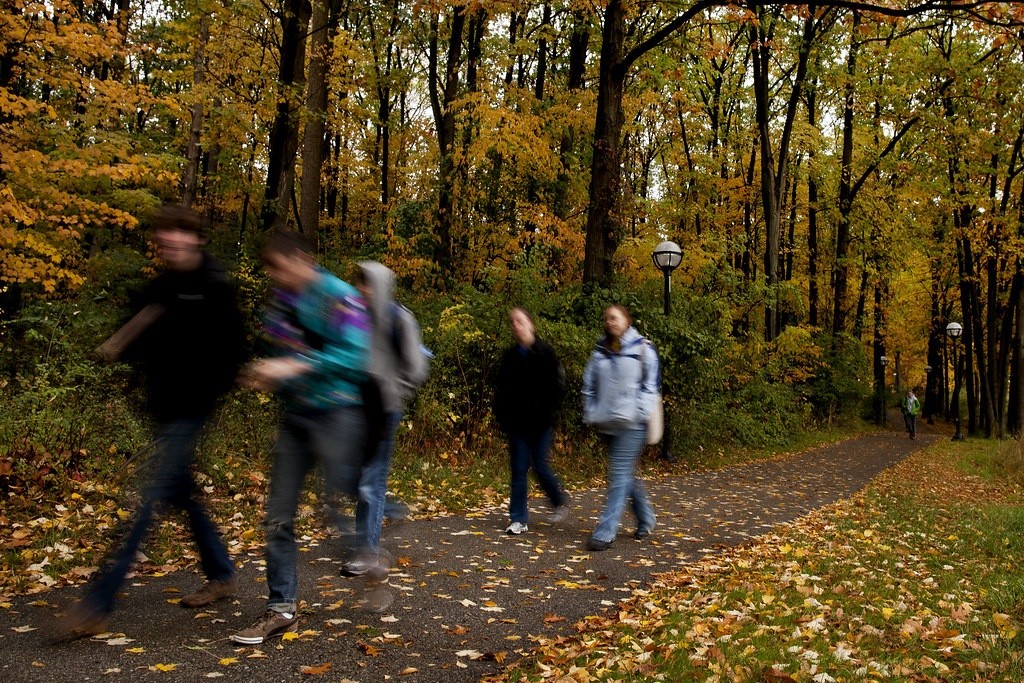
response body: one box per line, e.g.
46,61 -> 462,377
634,529 -> 651,538
555,491 -> 571,524
505,522 -> 529,535
340,555 -> 379,576
585,539 -> 615,550
229,609 -> 298,644
59,606 -> 112,635
180,577 -> 238,609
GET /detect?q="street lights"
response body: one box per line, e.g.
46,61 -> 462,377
649,240 -> 684,464
946,320 -> 965,442
880,355 -> 890,428
924,364 -> 933,425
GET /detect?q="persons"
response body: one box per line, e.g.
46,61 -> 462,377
231,232 -> 379,648
901,389 -> 921,440
576,303 -> 668,551
487,306 -> 571,535
340,261 -> 422,576
31,213 -> 250,639
